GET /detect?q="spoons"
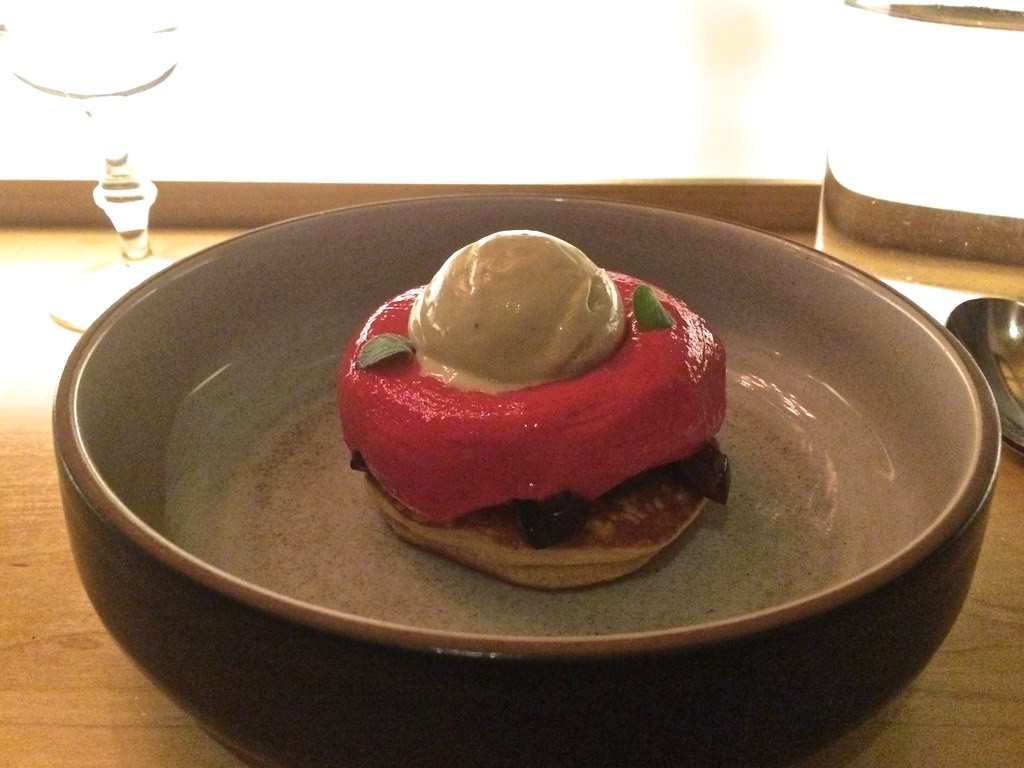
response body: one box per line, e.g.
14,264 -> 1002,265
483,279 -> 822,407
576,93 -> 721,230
947,297 -> 1024,459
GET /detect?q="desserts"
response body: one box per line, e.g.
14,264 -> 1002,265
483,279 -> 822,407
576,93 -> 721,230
340,229 -> 732,586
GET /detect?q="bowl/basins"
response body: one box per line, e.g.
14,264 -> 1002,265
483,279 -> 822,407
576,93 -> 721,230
53,194 -> 1002,767
